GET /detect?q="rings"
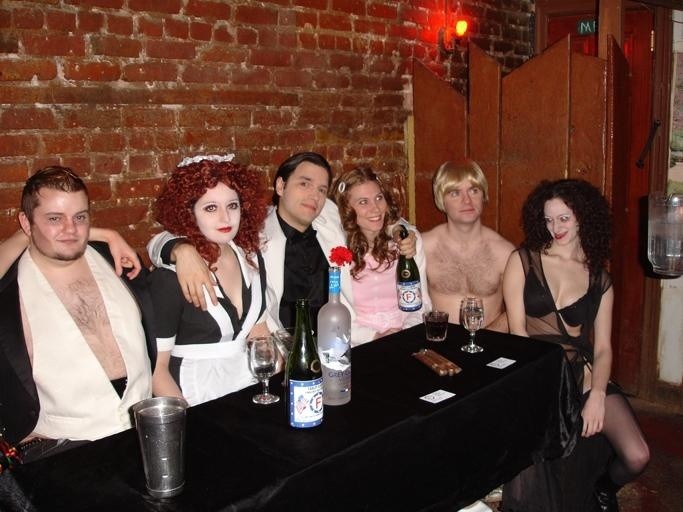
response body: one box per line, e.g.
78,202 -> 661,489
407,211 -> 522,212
413,236 -> 417,241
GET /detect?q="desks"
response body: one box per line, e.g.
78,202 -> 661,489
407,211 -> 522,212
10,320 -> 562,512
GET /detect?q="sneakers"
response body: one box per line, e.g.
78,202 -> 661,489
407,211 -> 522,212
592,470 -> 620,512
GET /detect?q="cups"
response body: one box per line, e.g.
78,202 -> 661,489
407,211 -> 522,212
131,396 -> 189,496
460,298 -> 485,354
423,309 -> 449,340
251,337 -> 279,404
276,330 -> 293,386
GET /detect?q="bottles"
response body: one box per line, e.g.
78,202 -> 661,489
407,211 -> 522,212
315,266 -> 351,406
395,229 -> 423,313
282,300 -> 324,430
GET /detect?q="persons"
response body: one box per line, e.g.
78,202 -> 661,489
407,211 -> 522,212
332,167 -> 432,349
0,167 -> 158,512
503,178 -> 649,512
141,155 -> 285,408
0,227 -> 141,279
147,153 -> 417,358
419,159 -> 518,504
